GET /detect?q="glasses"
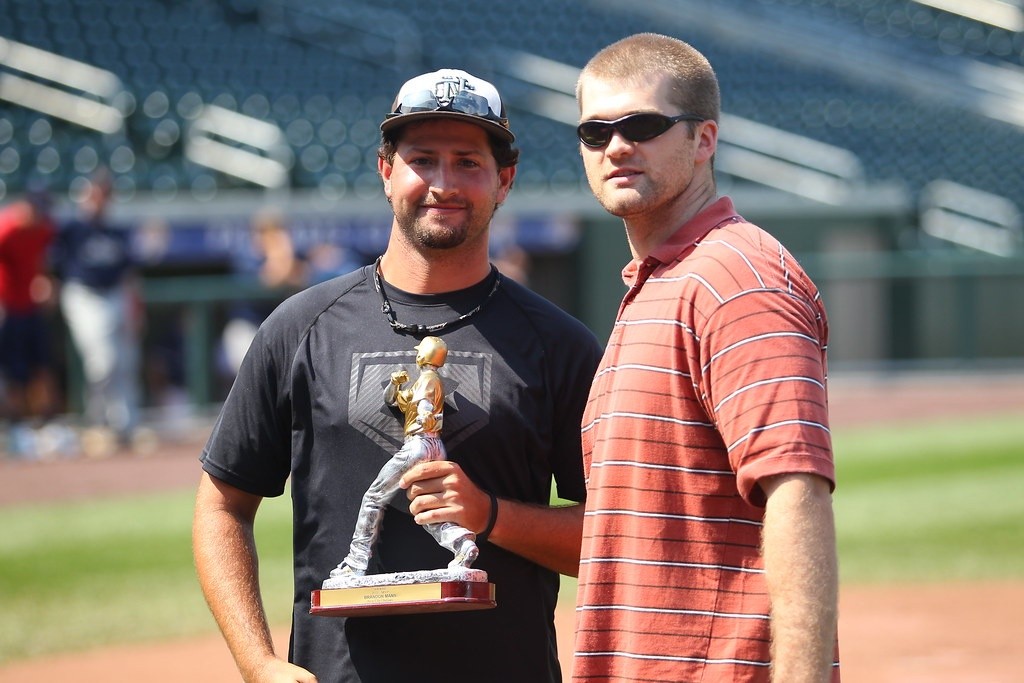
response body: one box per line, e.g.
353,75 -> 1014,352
576,112 -> 706,149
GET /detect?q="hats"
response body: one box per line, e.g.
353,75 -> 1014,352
381,68 -> 516,145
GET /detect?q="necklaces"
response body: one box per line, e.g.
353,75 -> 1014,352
372,250 -> 501,334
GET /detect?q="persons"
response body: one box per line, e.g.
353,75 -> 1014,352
191,68 -> 606,683
0,164 -> 525,454
574,32 -> 841,682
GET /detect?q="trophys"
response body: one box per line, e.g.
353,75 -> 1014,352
309,335 -> 498,618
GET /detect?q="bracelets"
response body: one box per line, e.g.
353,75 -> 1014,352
471,489 -> 499,545
